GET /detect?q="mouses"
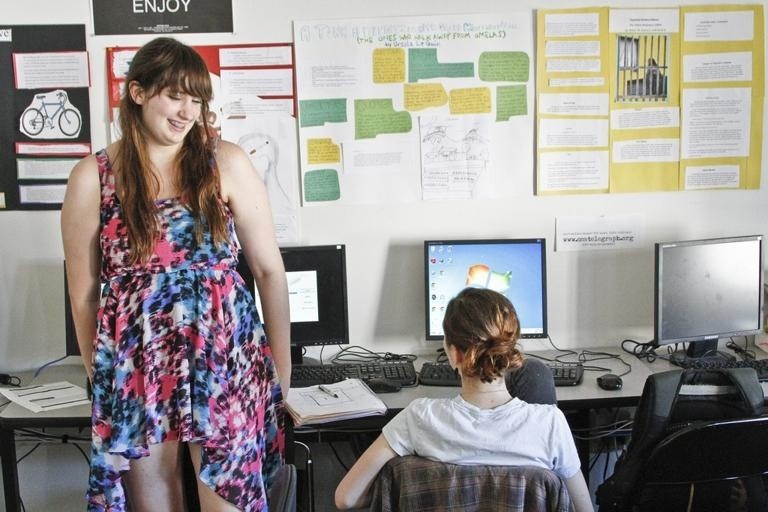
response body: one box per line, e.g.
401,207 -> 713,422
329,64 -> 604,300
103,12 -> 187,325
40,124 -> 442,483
597,374 -> 622,390
366,378 -> 402,393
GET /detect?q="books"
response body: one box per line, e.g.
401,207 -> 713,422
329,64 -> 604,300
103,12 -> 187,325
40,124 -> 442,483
279,377 -> 389,424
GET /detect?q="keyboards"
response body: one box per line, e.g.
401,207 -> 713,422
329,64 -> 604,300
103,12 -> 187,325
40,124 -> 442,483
689,359 -> 768,383
290,360 -> 416,388
418,362 -> 583,386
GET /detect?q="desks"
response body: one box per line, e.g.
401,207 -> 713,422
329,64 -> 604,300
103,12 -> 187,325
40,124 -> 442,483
0,351 -> 767,511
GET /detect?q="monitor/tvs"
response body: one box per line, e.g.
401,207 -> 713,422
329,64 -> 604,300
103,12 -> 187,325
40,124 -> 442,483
63,260 -> 81,356
235,244 -> 350,364
654,235 -> 765,367
424,238 -> 547,340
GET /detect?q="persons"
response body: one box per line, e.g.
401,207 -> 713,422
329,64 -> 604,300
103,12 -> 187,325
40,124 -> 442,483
60,35 -> 297,512
333,285 -> 598,512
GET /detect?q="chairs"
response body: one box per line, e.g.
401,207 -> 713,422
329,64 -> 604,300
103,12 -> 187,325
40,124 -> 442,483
371,458 -> 574,511
268,407 -> 316,510
595,375 -> 768,512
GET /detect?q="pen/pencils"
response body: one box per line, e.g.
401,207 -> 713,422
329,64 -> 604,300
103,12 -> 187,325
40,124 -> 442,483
319,385 -> 339,398
